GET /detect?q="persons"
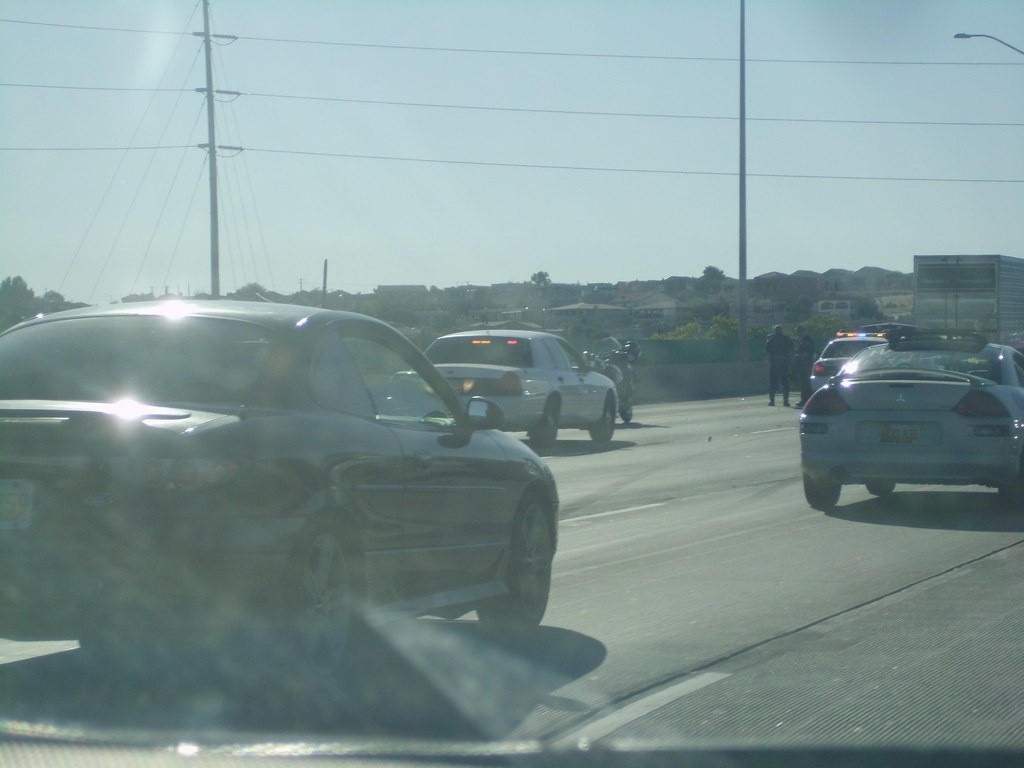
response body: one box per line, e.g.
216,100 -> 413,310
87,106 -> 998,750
764,325 -> 795,406
793,326 -> 816,405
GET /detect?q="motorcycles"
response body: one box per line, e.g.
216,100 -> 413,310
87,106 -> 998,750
582,336 -> 640,421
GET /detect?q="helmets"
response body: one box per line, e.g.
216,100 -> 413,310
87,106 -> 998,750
625,341 -> 641,358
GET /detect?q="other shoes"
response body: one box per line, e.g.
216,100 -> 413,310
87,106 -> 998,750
796,400 -> 805,405
783,401 -> 790,406
768,400 -> 775,406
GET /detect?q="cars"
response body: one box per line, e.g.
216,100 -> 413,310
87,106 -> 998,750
799,326 -> 1024,512
385,330 -> 621,448
808,331 -> 892,393
1,301 -> 558,681
